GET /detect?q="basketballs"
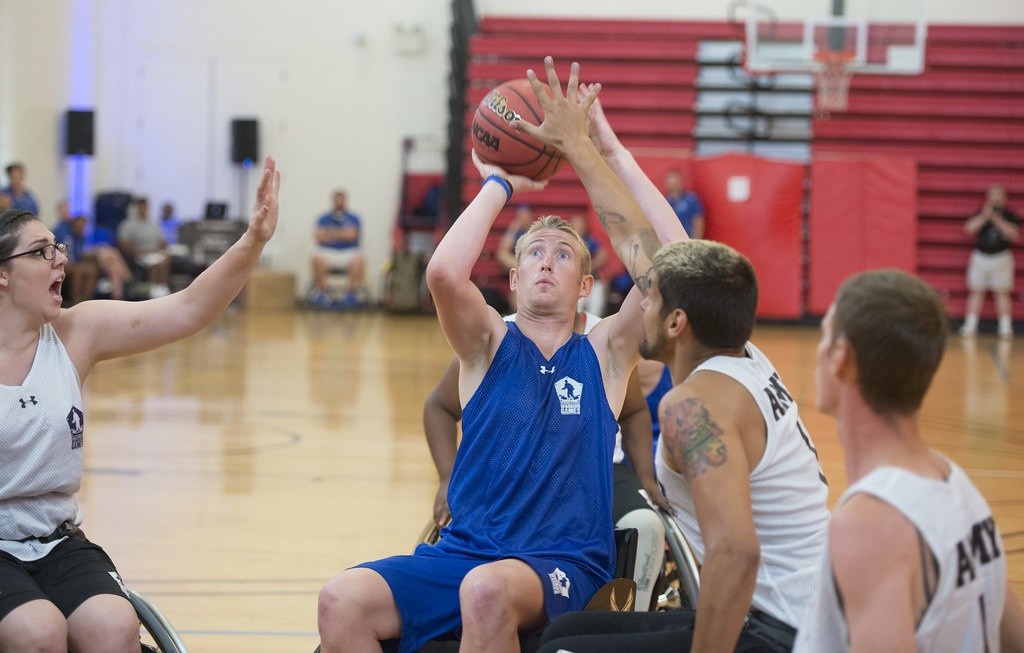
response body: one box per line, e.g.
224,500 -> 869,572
471,77 -> 565,181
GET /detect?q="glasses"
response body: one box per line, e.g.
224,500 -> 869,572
0,243 -> 68,261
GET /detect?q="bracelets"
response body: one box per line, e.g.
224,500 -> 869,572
489,173 -> 514,197
482,176 -> 511,201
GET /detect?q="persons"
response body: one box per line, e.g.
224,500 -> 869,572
311,193 -> 367,310
956,187 -> 1024,339
0,155 -> 282,653
792,270 -> 1024,653
318,56 -> 830,653
0,163 -> 190,307
